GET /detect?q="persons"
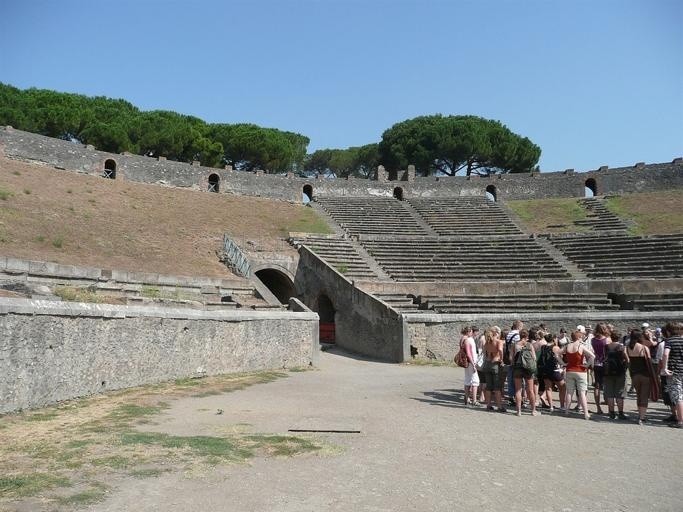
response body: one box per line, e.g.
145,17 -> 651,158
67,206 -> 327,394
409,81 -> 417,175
459,320 -> 683,428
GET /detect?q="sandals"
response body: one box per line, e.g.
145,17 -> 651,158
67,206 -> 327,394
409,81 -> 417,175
516,405 -> 592,420
638,416 -> 648,425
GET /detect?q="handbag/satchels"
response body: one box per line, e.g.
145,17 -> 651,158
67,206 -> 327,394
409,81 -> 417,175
474,351 -> 485,372
454,337 -> 469,368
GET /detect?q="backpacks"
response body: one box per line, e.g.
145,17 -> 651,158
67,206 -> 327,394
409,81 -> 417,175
515,341 -> 536,375
503,332 -> 519,365
537,344 -> 556,373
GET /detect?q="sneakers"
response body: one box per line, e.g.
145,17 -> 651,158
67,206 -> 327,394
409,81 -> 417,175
464,396 -> 529,413
609,413 -> 629,420
662,415 -> 683,428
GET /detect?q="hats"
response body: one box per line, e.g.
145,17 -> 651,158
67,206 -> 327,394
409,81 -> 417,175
577,324 -> 585,333
641,322 -> 649,329
